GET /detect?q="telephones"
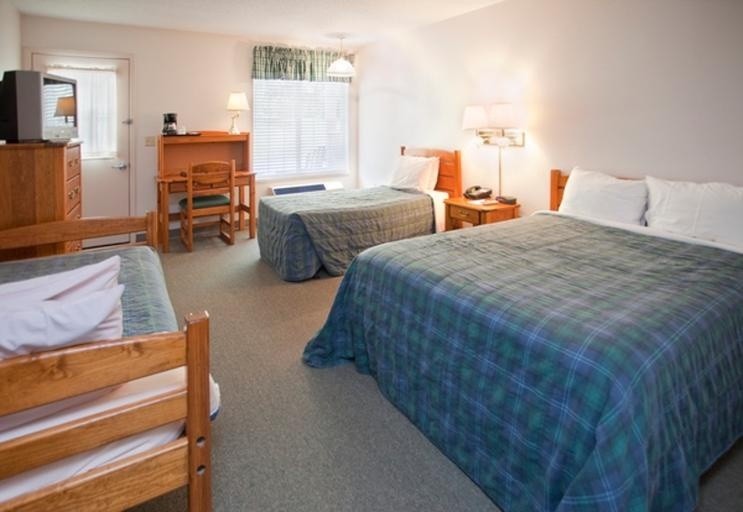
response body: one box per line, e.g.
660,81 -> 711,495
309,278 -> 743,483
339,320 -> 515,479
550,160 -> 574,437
464,186 -> 492,199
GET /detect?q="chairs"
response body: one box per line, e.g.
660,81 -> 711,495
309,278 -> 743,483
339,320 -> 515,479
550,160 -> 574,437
179,159 -> 235,251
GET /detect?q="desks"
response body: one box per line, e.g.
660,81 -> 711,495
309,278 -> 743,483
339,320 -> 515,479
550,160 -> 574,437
155,130 -> 256,253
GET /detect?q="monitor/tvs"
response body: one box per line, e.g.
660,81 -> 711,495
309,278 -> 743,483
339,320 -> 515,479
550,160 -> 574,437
0,69 -> 80,143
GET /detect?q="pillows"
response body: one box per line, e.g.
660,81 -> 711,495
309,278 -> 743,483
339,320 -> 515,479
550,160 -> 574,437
0,366 -> 219,502
646,175 -> 743,249
558,167 -> 646,226
390,157 -> 439,191
0,256 -> 124,430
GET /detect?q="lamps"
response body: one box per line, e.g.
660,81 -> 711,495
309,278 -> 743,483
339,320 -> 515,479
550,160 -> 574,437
463,103 -> 517,204
328,34 -> 355,76
54,97 -> 76,123
226,92 -> 251,135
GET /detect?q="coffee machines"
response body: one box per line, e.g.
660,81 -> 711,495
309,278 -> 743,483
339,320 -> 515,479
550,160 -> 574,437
159,114 -> 178,137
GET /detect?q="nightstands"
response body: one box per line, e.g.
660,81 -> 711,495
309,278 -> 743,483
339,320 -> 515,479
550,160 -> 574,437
444,197 -> 521,230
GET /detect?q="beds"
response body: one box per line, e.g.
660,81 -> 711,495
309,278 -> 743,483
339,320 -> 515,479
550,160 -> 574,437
257,146 -> 461,282
0,211 -> 219,512
302,170 -> 743,512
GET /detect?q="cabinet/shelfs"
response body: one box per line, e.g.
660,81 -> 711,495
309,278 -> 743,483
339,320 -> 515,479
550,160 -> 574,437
0,141 -> 82,260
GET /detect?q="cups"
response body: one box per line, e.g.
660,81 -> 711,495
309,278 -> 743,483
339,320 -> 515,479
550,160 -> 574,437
175,129 -> 199,135
